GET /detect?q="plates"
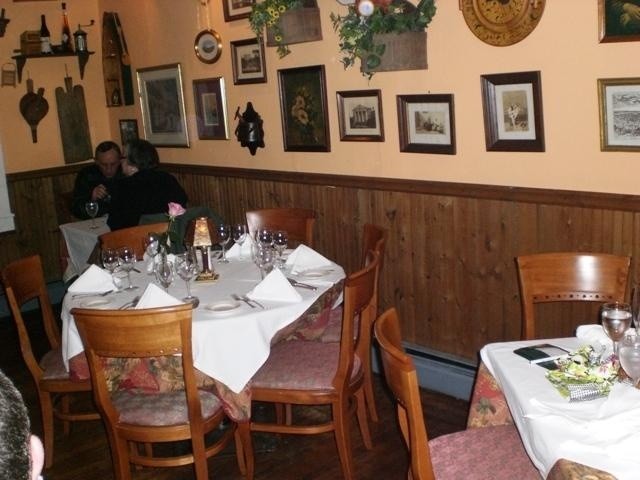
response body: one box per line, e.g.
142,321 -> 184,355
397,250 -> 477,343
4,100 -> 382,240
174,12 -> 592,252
297,269 -> 330,278
204,299 -> 241,312
81,297 -> 115,309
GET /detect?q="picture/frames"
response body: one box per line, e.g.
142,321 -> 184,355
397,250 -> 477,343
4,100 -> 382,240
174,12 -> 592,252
597,77 -> 640,152
335,89 -> 386,142
396,93 -> 458,156
479,69 -> 546,154
191,75 -> 230,141
230,36 -> 267,85
277,64 -> 331,152
597,0 -> 640,44
136,62 -> 190,150
222,0 -> 257,24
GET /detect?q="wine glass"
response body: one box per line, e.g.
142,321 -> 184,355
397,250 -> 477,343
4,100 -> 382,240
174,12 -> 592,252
101,232 -> 201,308
217,225 -> 287,281
601,300 -> 640,389
86,202 -> 99,229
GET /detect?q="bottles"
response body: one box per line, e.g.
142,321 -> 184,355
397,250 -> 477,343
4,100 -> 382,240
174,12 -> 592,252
40,3 -> 87,56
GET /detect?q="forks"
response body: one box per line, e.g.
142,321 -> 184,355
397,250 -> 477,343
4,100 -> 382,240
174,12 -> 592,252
72,290 -> 112,297
118,296 -> 140,309
231,293 -> 264,309
286,278 -> 317,291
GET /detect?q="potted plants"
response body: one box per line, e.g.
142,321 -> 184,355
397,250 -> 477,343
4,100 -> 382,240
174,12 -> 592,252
329,1 -> 436,80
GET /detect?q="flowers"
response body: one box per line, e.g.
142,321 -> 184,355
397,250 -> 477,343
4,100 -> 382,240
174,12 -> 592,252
248,0 -> 301,58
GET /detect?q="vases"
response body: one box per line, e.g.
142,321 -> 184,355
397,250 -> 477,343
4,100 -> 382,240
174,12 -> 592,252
267,0 -> 323,48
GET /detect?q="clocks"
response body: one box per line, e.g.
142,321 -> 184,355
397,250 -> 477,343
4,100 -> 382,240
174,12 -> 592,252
194,1 -> 223,64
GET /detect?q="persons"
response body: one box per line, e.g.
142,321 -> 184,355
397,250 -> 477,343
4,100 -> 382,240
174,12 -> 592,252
0,368 -> 45,479
69,141 -> 127,219
107,139 -> 190,230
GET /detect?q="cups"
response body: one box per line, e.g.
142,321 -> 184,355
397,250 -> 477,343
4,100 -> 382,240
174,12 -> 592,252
195,247 -> 212,270
